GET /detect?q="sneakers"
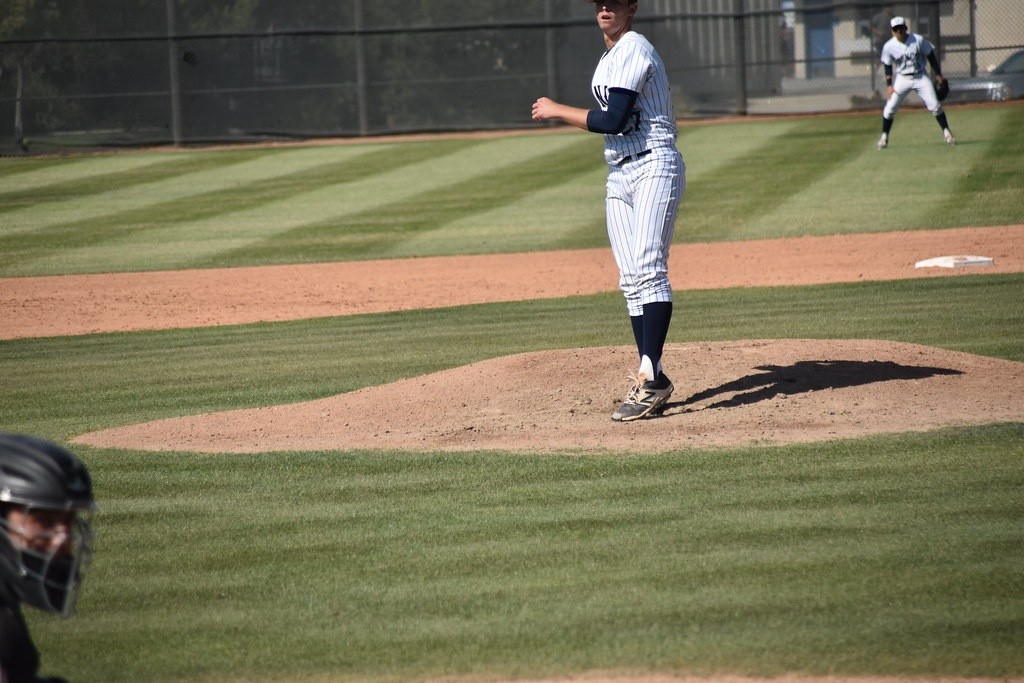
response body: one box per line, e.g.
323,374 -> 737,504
610,368 -> 674,422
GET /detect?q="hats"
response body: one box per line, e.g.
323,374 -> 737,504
890,16 -> 905,28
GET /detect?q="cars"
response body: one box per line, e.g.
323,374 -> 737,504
987,50 -> 1024,102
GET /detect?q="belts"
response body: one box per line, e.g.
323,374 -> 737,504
622,150 -> 651,160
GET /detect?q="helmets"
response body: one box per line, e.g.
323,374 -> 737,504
0,434 -> 96,612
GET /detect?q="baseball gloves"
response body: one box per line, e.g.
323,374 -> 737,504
938,79 -> 949,99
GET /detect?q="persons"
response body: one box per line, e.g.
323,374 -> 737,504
532,0 -> 687,421
0,434 -> 95,683
878,16 -> 956,149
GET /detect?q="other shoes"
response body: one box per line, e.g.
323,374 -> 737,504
944,134 -> 955,144
877,139 -> 887,150
